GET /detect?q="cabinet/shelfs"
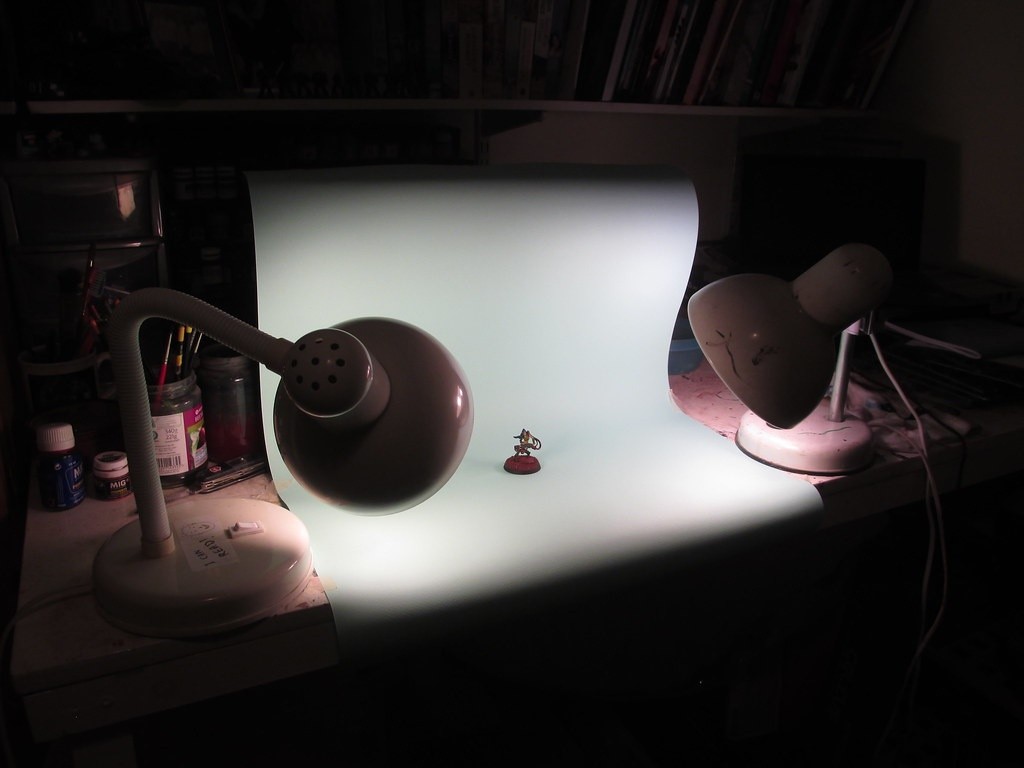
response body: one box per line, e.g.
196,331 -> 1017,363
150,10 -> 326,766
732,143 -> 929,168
1,100 -> 887,412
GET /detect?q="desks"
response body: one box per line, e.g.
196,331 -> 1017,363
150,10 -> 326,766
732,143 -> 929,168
10,361 -> 1023,767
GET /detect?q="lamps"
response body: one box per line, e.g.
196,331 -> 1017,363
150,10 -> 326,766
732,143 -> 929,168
94,287 -> 476,641
688,244 -> 905,476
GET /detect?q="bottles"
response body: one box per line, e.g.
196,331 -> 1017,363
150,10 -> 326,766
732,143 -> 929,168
32,423 -> 87,514
200,345 -> 263,464
91,452 -> 132,501
138,364 -> 210,488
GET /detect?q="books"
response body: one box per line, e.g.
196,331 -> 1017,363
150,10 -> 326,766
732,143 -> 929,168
0,0 -> 917,110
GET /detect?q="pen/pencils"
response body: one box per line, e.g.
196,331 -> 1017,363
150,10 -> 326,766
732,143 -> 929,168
152,324 -> 204,415
